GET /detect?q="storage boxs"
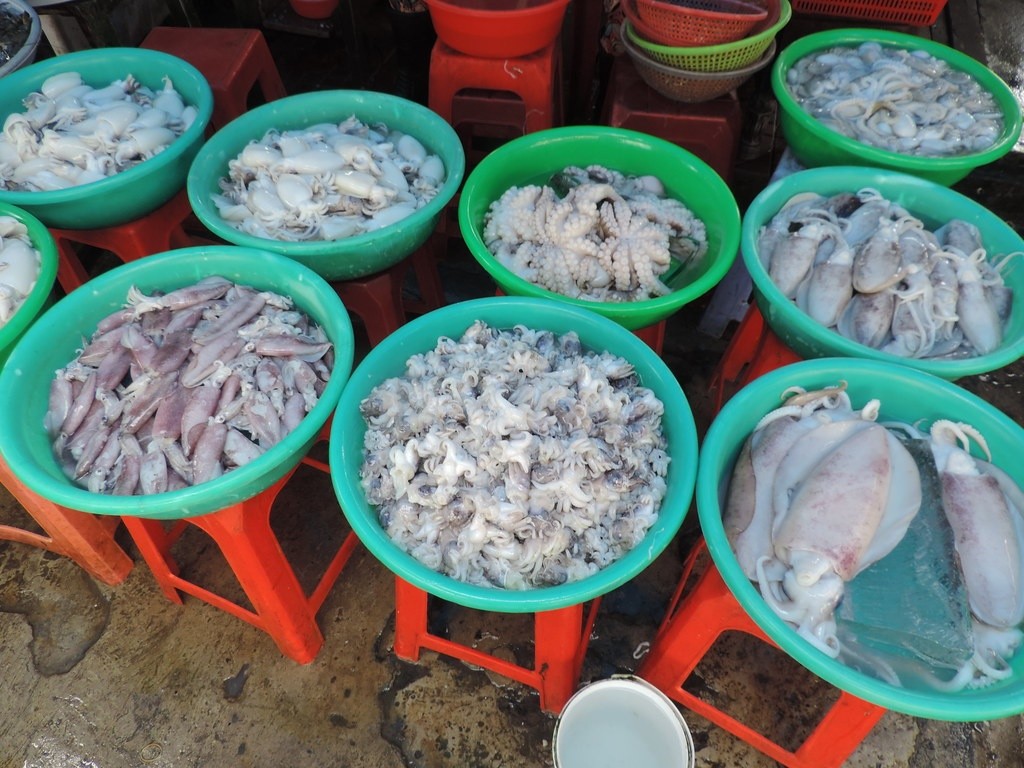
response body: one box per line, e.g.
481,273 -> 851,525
792,0 -> 949,26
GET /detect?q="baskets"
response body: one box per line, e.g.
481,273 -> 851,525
791,0 -> 947,28
621,0 -> 794,104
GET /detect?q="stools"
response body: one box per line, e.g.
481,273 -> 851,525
0,26 -> 937,768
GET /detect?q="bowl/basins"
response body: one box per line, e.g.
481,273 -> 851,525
0,245 -> 355,520
0,201 -> 59,374
328,294 -> 699,613
696,357 -> 1024,722
740,166 -> 1024,382
0,0 -> 41,79
458,124 -> 742,333
0,47 -> 214,231
185,88 -> 466,282
424,0 -> 570,59
771,26 -> 1023,189
